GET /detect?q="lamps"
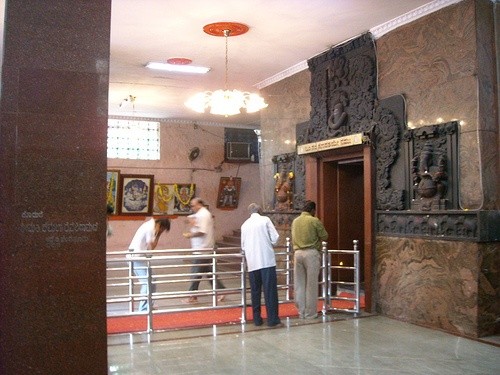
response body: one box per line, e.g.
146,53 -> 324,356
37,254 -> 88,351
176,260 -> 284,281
144,62 -> 211,74
186,22 -> 269,117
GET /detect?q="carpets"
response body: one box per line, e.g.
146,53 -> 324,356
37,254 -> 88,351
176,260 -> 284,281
107,292 -> 366,334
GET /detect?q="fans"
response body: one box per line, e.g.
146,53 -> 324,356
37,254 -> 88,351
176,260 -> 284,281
188,146 -> 200,161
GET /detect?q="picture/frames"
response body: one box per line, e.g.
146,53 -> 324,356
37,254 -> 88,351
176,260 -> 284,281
106,170 -> 196,216
216,177 -> 241,209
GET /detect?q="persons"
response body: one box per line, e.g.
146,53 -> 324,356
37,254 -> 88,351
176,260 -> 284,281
106,200 -> 116,238
239,202 -> 281,327
180,197 -> 228,304
290,200 -> 329,320
124,214 -> 172,312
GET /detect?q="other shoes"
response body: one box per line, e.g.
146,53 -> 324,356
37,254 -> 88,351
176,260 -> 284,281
181,297 -> 197,303
217,293 -> 224,303
274,322 -> 284,327
